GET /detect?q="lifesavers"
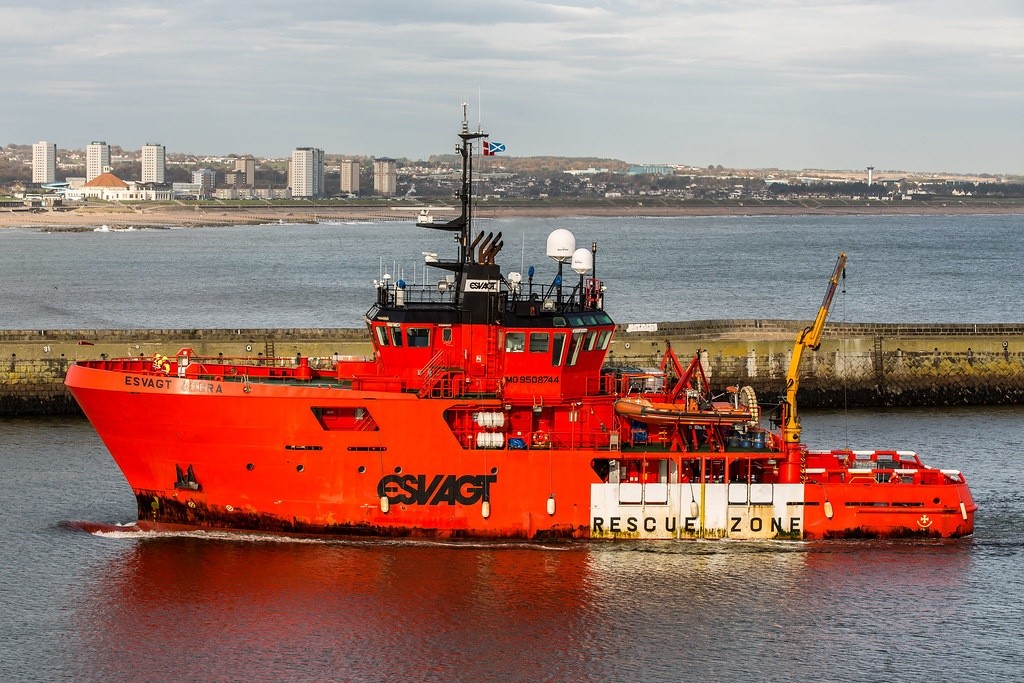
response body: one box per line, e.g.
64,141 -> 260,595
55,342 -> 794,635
888,475 -> 902,483
533,430 -> 548,445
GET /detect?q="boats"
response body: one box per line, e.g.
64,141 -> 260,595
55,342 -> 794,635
64,97 -> 979,542
614,396 -> 753,428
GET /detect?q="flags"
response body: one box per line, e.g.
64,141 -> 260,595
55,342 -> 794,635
483,141 -> 494,155
490,142 -> 505,152
